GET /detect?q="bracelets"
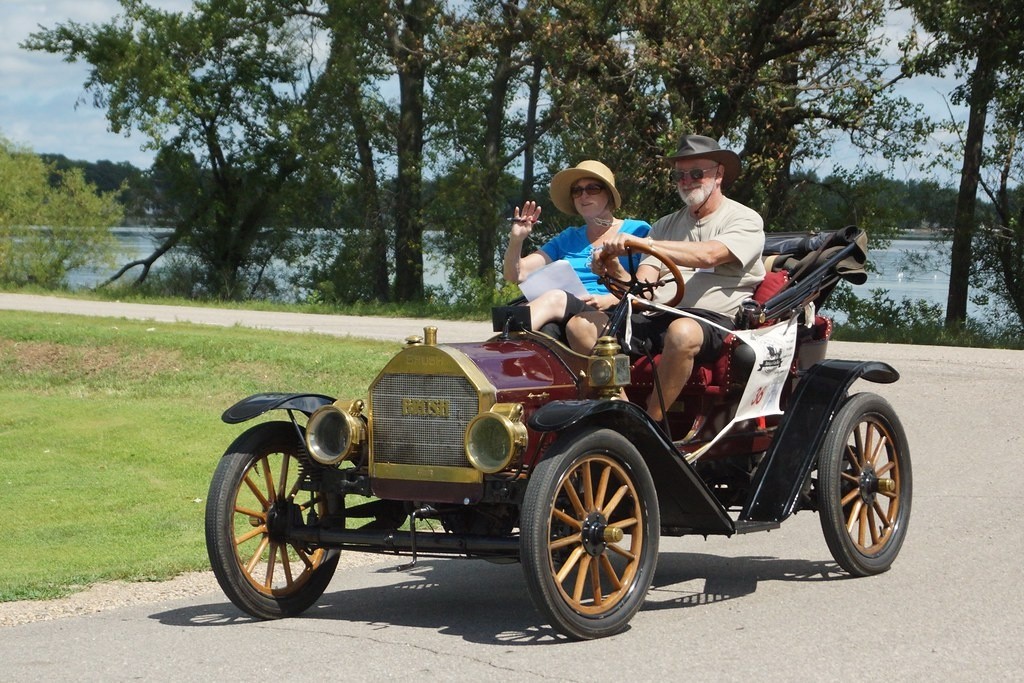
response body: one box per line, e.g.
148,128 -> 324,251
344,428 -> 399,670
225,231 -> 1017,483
644,234 -> 653,248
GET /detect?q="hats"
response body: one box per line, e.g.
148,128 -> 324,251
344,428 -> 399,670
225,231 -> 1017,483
550,160 -> 621,216
655,135 -> 741,193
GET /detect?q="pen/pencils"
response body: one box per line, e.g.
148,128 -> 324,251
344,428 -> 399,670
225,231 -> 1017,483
507,218 -> 542,224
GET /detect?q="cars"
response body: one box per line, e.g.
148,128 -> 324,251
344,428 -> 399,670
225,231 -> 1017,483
205,225 -> 912,641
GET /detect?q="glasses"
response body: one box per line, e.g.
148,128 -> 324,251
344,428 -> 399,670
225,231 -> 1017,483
669,165 -> 719,184
570,183 -> 608,199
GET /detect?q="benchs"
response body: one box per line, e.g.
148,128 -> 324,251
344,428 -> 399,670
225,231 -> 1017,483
495,225 -> 869,429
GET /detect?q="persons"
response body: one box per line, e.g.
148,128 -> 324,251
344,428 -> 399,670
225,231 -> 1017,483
500,159 -> 651,343
566,132 -> 767,433
